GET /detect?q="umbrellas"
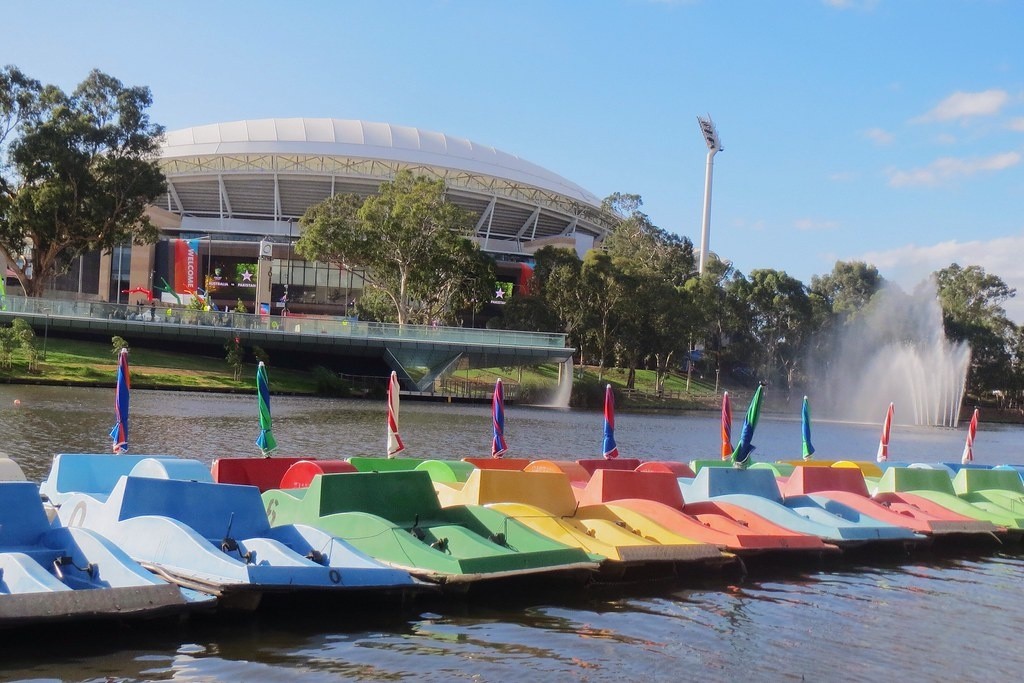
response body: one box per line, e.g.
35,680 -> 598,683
801,395 -> 817,460
387,371 -> 404,458
255,360 -> 278,458
491,377 -> 508,458
876,401 -> 893,462
729,379 -> 767,470
961,406 -> 980,465
602,384 -> 619,459
110,348 -> 132,454
721,391 -> 735,462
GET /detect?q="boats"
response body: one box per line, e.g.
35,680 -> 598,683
0,458 -> 1023,632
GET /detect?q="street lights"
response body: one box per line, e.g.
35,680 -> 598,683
42,307 -> 51,359
697,112 -> 724,272
285,218 -> 293,312
206,234 -> 211,311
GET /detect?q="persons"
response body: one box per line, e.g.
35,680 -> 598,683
150,303 -> 156,321
